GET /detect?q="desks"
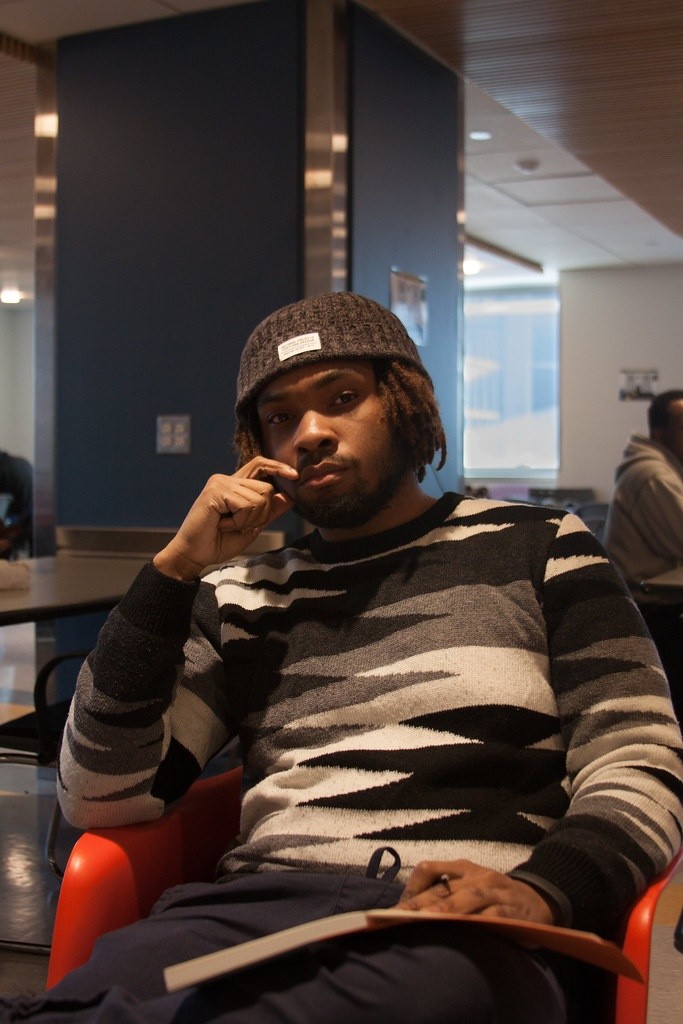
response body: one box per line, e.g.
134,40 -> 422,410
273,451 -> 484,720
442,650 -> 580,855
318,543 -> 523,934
0,555 -> 149,629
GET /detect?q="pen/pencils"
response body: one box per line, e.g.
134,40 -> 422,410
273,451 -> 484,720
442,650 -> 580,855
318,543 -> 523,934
442,874 -> 449,883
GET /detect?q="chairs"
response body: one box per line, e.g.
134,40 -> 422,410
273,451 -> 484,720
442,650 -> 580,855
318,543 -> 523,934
44,766 -> 683,1024
0,648 -> 90,956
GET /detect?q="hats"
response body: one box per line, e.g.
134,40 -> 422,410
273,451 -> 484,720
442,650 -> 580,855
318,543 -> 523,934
234,290 -> 433,422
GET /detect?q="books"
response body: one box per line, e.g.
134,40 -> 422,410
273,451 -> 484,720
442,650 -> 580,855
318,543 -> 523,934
163,908 -> 645,992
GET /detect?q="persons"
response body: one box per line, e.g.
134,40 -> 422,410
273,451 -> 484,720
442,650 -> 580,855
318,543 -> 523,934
0,295 -> 683,1024
605,390 -> 683,731
0,451 -> 33,561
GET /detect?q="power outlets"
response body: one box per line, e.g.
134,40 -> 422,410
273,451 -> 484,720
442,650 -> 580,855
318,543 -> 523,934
155,415 -> 191,453
